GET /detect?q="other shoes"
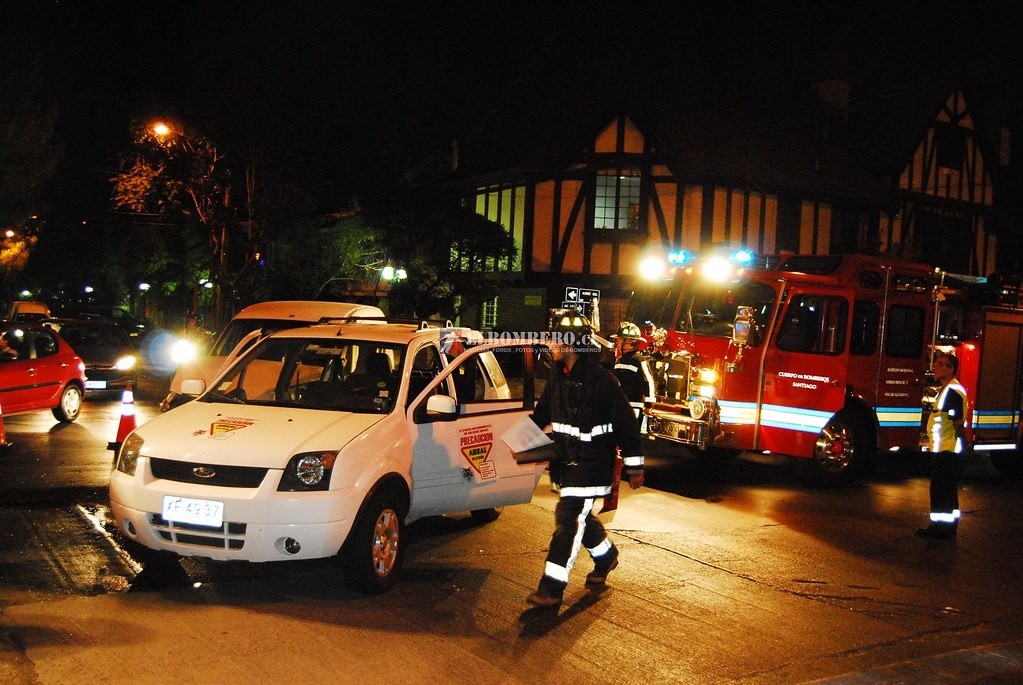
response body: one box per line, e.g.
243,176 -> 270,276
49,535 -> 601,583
585,557 -> 618,583
914,521 -> 957,541
526,587 -> 563,605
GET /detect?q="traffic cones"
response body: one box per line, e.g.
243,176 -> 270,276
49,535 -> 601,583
105,380 -> 138,450
0,403 -> 13,448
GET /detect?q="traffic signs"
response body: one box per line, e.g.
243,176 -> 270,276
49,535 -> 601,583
561,301 -> 585,315
565,287 -> 600,304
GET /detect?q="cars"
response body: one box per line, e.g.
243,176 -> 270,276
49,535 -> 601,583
159,301 -> 396,415
62,318 -> 164,400
0,302 -> 87,423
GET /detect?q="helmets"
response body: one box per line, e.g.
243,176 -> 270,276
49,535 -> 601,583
610,322 -> 648,343
546,309 -> 592,347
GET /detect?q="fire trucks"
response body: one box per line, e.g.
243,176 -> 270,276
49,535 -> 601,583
612,243 -> 1023,493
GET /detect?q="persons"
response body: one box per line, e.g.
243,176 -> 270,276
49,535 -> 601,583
32,333 -> 54,358
0,331 -> 20,359
915,343 -> 972,542
511,326 -> 644,611
608,321 -> 651,433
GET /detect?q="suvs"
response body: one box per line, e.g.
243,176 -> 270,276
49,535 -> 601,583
109,316 -> 558,594
